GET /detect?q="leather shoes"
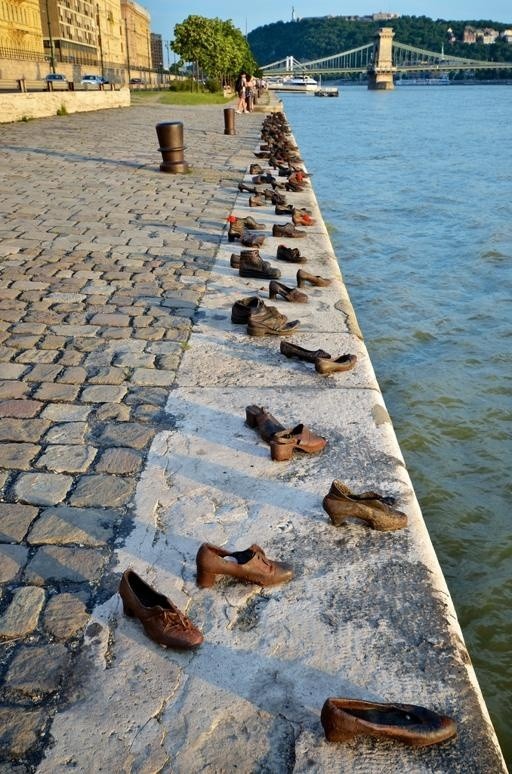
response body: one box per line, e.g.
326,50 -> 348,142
280,341 -> 331,363
314,353 -> 358,374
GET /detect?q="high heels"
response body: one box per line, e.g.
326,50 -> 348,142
322,478 -> 408,532
269,423 -> 327,461
297,269 -> 331,288
245,404 -> 287,443
272,222 -> 306,238
118,568 -> 205,651
320,696 -> 459,747
195,542 -> 294,589
268,280 -> 308,303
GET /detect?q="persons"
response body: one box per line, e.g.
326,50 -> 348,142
235,71 -> 268,115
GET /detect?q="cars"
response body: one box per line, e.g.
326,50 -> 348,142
131,78 -> 145,84
46,74 -> 63,81
81,74 -> 107,85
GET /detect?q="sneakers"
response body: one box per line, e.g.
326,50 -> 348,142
246,310 -> 300,337
230,254 -> 241,268
292,207 -> 314,227
231,296 -> 287,325
238,110 -> 313,208
228,215 -> 266,248
275,204 -> 294,215
277,245 -> 306,263
239,250 -> 281,279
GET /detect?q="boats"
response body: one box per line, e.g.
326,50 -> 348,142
285,76 -> 317,85
314,88 -> 337,97
